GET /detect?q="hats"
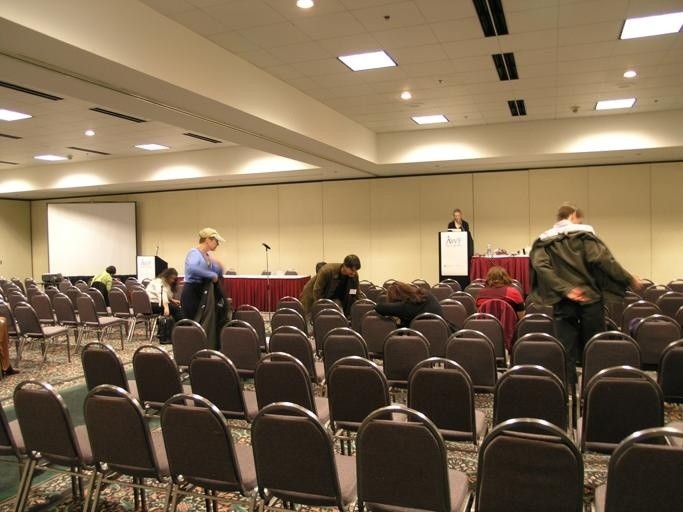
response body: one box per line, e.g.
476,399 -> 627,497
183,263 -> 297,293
199,228 -> 225,242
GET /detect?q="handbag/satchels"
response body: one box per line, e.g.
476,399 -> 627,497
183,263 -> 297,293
158,316 -> 175,344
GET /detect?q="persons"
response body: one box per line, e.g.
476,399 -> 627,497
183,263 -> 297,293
300,254 -> 362,314
144,266 -> 185,326
446,209 -> 469,232
375,278 -> 460,335
315,262 -> 326,273
178,228 -> 225,334
524,203 -> 647,361
88,265 -> 117,313
473,267 -> 524,321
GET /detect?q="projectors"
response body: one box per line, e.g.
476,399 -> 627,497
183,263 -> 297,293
41,272 -> 62,282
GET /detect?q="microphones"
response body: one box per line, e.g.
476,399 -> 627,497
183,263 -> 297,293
155,246 -> 159,256
262,242 -> 272,251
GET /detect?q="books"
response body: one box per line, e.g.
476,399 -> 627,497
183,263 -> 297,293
448,228 -> 461,232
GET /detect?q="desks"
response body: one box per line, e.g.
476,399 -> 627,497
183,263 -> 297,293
468,253 -> 533,295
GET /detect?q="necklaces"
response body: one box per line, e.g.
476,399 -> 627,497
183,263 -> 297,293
198,249 -> 211,267
